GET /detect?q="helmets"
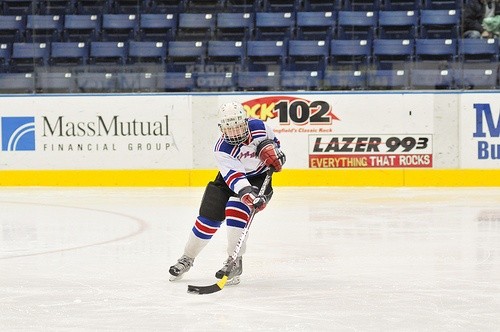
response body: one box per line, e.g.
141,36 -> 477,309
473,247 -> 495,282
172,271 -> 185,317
216,102 -> 246,127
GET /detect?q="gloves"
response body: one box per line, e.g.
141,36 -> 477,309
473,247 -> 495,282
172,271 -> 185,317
238,186 -> 266,212
257,141 -> 283,173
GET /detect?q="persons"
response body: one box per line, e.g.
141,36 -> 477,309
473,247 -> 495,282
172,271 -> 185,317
169,102 -> 286,279
436,61 -> 474,90
464,0 -> 500,40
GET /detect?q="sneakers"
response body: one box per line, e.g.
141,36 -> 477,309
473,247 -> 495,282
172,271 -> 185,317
216,256 -> 242,285
169,255 -> 193,281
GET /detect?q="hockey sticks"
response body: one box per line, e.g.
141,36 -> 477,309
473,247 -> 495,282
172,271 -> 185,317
188,165 -> 275,295
